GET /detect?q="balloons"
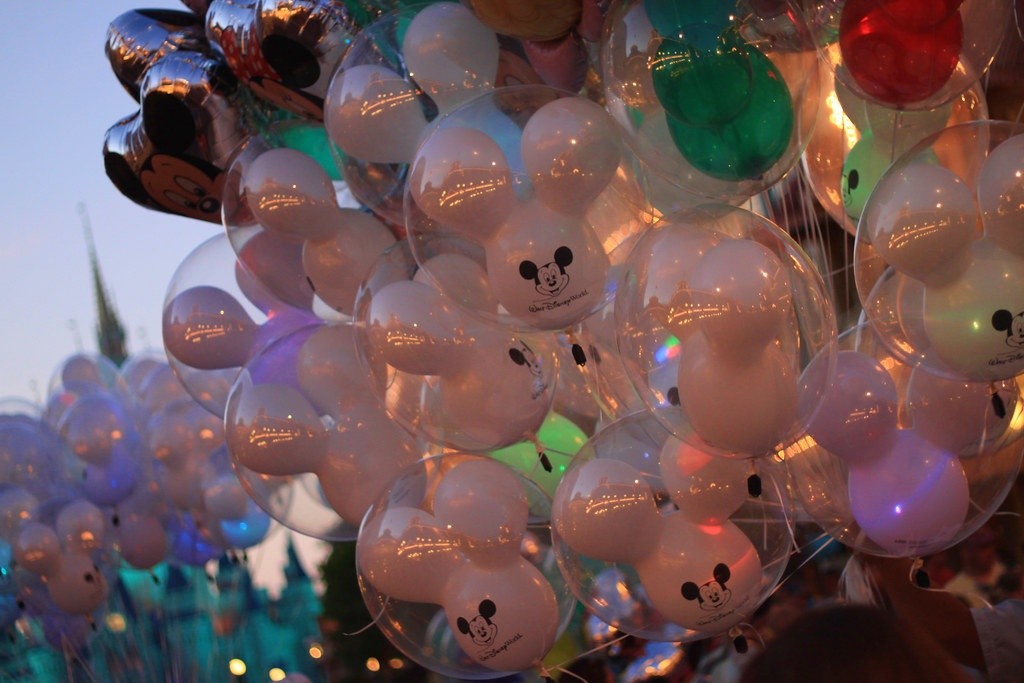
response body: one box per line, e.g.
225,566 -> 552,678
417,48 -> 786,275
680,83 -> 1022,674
0,354 -> 303,655
100,0 -> 1024,682
